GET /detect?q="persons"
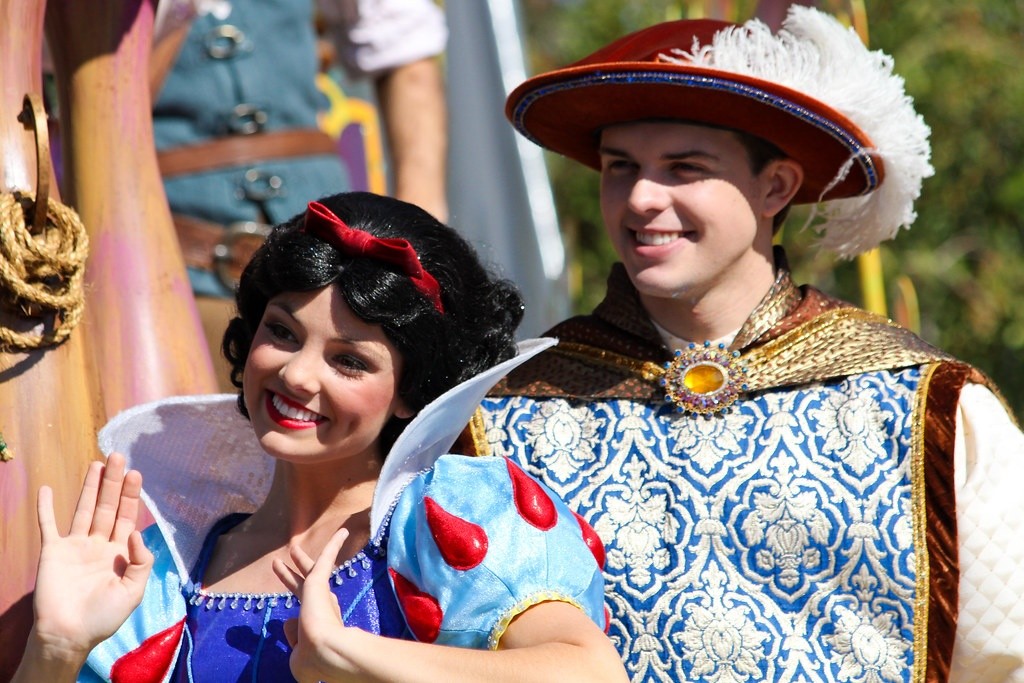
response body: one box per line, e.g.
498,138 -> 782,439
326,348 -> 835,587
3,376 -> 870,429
0,191 -> 633,683
42,0 -> 451,391
448,3 -> 1024,683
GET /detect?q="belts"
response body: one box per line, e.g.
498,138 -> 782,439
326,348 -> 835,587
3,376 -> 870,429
155,130 -> 340,291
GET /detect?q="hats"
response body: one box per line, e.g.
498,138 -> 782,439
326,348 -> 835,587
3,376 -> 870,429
505,2 -> 937,261
